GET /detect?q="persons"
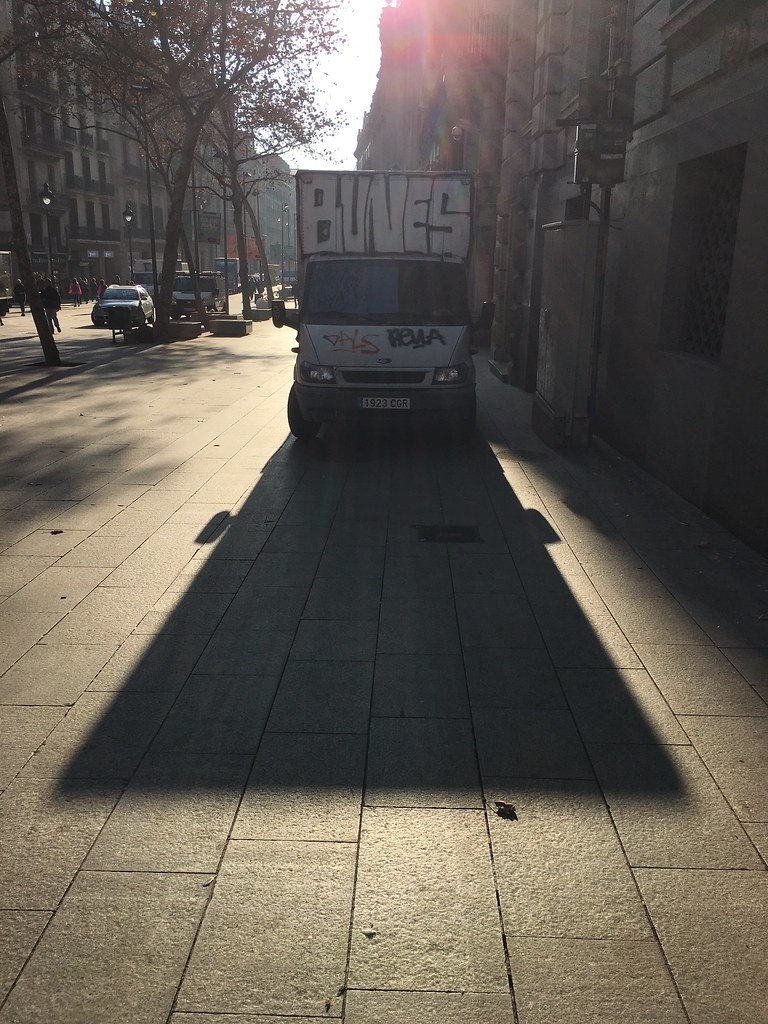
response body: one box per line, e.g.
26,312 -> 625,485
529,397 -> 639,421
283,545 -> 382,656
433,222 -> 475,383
292,280 -> 299,309
0,271 -> 135,334
248,277 -> 266,301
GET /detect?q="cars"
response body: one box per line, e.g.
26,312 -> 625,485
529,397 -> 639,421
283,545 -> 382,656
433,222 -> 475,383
91,285 -> 155,328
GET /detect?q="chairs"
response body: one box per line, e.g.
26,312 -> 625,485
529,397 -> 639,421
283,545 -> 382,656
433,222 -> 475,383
107,308 -> 132,343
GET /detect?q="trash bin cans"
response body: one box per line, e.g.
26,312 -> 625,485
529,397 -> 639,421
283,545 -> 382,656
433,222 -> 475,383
255,293 -> 263,304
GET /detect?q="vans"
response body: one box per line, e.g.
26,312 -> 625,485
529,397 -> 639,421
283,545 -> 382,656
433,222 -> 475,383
169,270 -> 225,318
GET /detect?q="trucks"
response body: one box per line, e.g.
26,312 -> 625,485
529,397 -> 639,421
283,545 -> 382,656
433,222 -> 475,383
127,259 -> 165,299
216,258 -> 241,294
271,169 -> 497,443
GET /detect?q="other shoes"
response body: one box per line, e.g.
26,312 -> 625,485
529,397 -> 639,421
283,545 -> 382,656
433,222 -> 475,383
74,305 -> 76,307
78,305 -> 80,307
52,330 -> 54,334
21,314 -> 25,316
85,302 -> 88,304
58,328 -> 61,332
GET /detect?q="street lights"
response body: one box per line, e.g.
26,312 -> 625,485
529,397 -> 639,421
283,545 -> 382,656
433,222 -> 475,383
252,188 -> 262,295
279,201 -> 289,301
240,170 -> 253,246
122,204 -> 137,283
38,182 -> 55,291
212,152 -> 230,315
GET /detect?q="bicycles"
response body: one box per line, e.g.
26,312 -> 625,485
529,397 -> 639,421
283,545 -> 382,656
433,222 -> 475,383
273,287 -> 281,300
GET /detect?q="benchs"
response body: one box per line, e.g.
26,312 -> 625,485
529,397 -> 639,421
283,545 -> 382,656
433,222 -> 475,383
169,309 -> 273,340
